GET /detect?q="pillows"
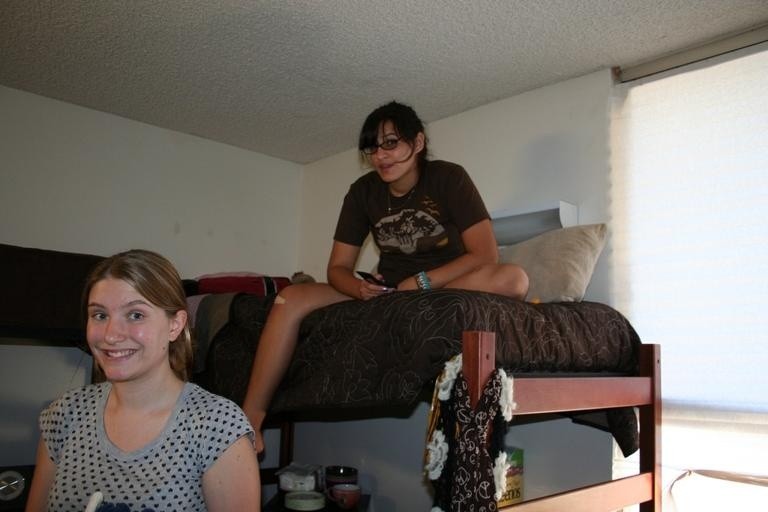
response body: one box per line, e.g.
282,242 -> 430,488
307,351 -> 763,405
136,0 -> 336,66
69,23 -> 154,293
496,222 -> 608,302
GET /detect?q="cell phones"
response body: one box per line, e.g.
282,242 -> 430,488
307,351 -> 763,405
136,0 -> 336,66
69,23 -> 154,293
355,271 -> 394,288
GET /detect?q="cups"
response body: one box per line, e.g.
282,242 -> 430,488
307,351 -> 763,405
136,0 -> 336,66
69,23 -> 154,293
327,483 -> 361,509
325,465 -> 357,500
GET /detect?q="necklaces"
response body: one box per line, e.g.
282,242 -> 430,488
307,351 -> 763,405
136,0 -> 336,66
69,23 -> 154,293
386,186 -> 416,213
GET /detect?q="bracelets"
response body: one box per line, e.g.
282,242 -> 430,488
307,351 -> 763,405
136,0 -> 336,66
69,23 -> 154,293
416,269 -> 430,291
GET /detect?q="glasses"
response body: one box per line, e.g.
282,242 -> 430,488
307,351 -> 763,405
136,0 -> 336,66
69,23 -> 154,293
363,136 -> 402,154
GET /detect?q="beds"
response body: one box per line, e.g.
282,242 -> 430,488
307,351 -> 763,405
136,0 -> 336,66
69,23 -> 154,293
172,272 -> 664,423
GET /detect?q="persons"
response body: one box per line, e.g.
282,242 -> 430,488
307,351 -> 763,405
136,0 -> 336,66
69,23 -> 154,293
24,249 -> 262,512
239,100 -> 530,454
